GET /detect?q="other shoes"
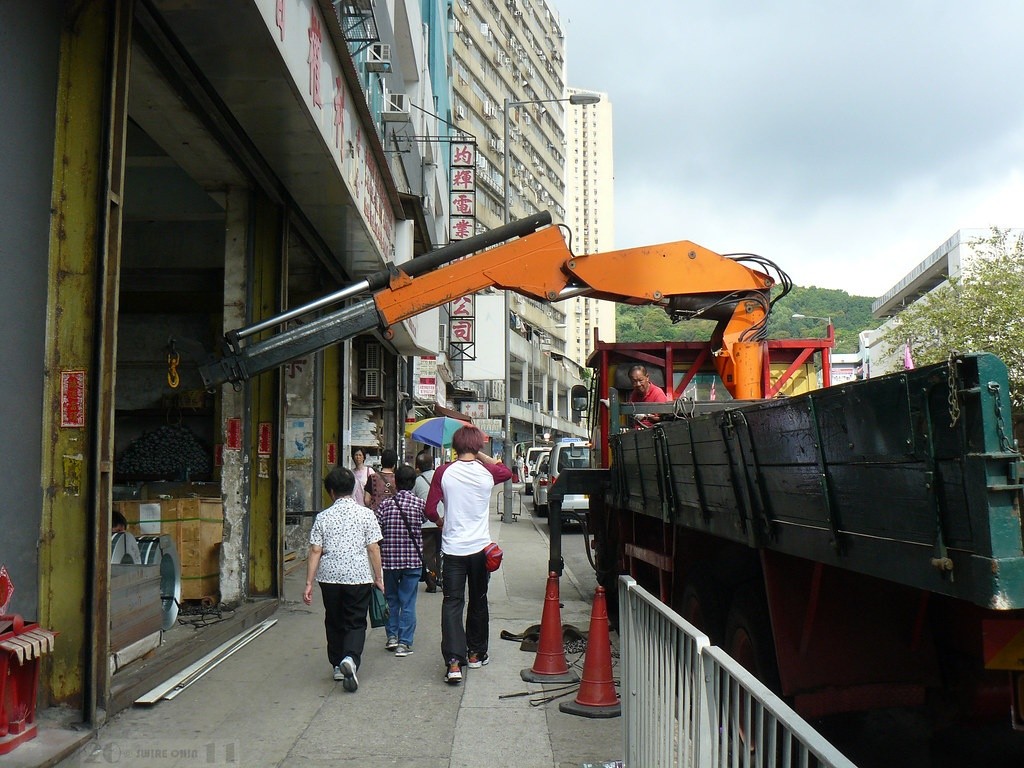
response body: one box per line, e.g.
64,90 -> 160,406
385,636 -> 398,648
340,656 -> 358,691
426,587 -> 436,592
396,644 -> 413,656
333,666 -> 344,680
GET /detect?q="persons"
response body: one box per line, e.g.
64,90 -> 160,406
425,425 -> 513,683
111,510 -> 127,531
375,465 -> 428,657
303,467 -> 383,692
515,454 -> 526,483
627,365 -> 667,428
350,444 -> 444,593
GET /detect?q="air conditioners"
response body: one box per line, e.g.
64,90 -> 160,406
517,130 -> 522,136
513,168 -> 518,176
548,201 -> 554,206
370,44 -> 393,72
491,108 -> 496,116
457,106 -> 465,118
537,184 -> 543,191
466,38 -> 472,46
386,93 -> 411,112
498,140 -> 505,154
366,344 -> 380,397
540,55 -> 546,61
515,11 -> 522,17
532,157 -> 538,163
484,101 -> 490,116
513,70 -> 520,78
490,139 -> 495,148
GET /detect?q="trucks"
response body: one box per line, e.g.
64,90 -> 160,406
526,323 -> 1024,768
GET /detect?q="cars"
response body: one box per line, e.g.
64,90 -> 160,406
529,452 -> 550,517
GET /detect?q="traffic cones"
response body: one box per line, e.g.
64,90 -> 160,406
520,570 -> 581,684
559,584 -> 621,719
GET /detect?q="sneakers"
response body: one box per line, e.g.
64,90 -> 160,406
468,650 -> 489,667
444,658 -> 462,683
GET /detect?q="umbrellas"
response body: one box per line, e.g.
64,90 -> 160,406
405,416 -> 489,465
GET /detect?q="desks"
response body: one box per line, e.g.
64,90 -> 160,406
112,497 -> 225,599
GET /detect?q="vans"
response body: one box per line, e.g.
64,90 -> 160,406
522,447 -> 553,496
544,441 -> 590,528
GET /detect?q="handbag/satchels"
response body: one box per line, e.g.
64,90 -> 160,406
369,585 -> 390,628
418,560 -> 426,582
484,543 -> 503,572
